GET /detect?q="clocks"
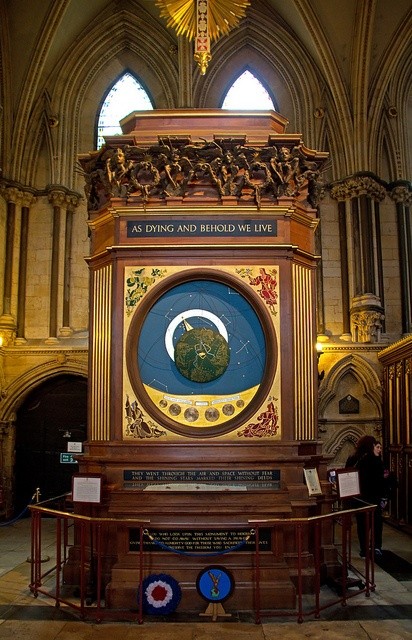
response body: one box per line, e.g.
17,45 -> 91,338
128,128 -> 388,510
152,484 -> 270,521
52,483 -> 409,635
195,565 -> 235,603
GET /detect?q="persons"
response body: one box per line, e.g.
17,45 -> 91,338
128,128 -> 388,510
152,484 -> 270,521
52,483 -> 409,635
355,441 -> 384,559
